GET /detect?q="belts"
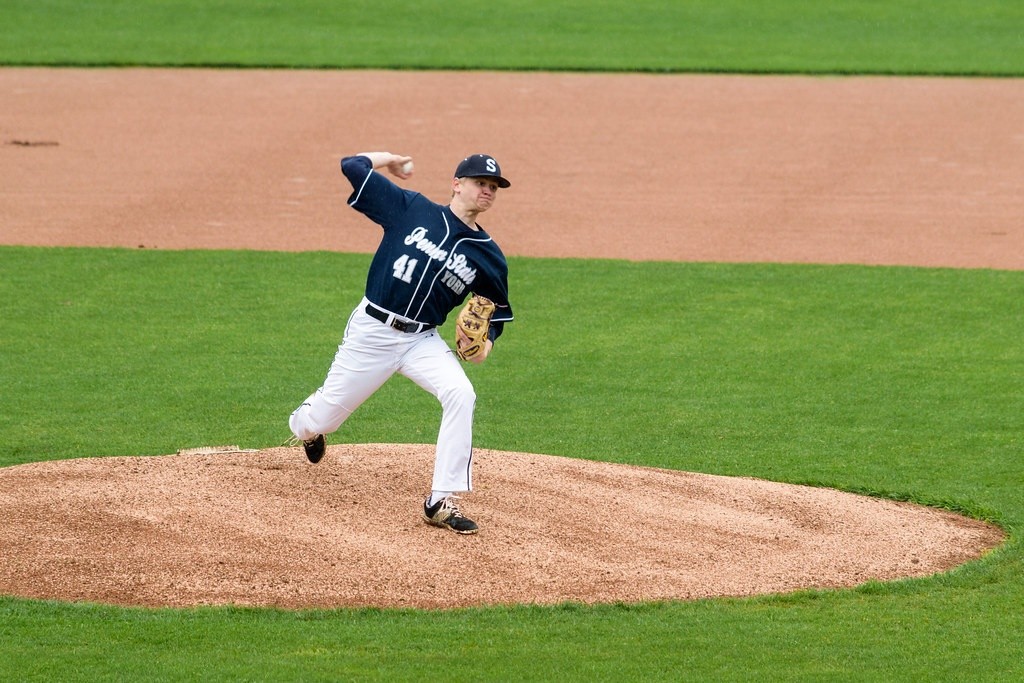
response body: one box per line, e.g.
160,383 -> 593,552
365,304 -> 434,334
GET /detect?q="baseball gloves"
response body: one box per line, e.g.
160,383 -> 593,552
454,295 -> 496,362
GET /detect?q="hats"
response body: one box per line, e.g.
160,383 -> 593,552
455,154 -> 511,188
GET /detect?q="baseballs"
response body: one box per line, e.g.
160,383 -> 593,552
402,161 -> 414,174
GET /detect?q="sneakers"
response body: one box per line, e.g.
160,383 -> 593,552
421,491 -> 478,534
283,430 -> 326,464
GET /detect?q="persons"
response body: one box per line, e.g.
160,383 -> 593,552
288,151 -> 514,535
